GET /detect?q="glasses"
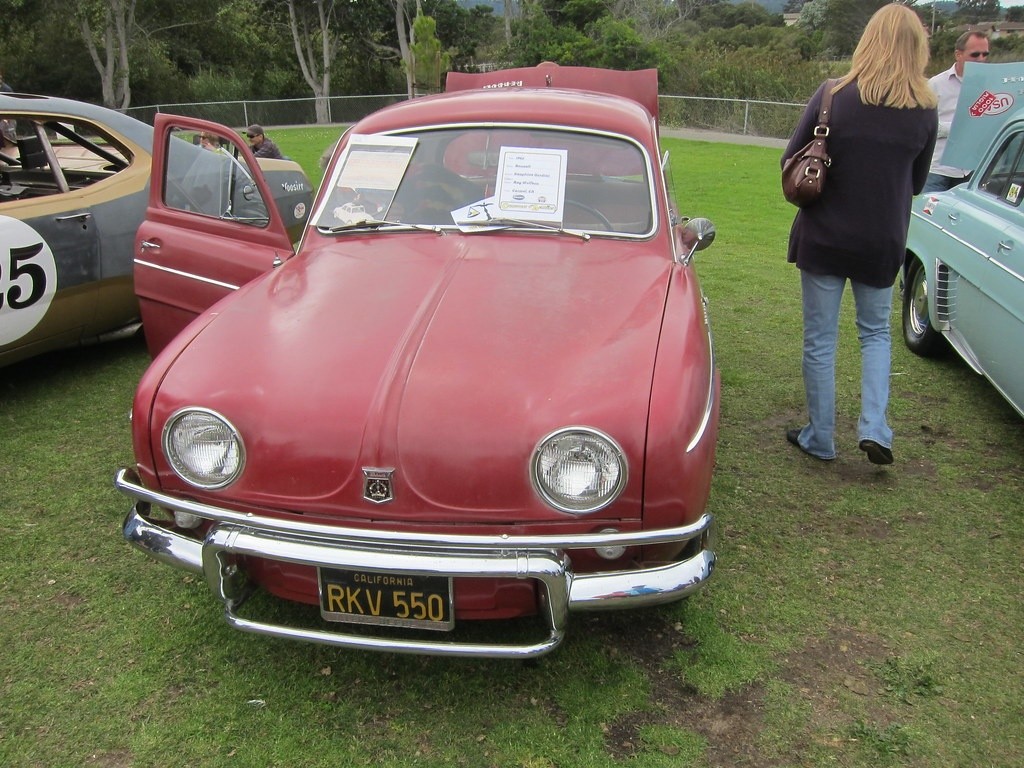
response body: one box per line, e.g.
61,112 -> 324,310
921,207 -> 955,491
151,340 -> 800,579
199,136 -> 207,139
970,51 -> 990,57
247,134 -> 254,138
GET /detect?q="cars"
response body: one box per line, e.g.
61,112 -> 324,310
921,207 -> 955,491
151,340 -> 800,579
117,61 -> 717,657
899,59 -> 1024,417
1,88 -> 315,367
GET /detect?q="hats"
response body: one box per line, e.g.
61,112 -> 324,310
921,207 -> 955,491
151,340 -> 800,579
242,125 -> 264,136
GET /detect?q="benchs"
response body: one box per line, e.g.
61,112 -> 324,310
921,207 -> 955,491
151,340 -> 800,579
469,178 -> 649,230
984,178 -> 1023,202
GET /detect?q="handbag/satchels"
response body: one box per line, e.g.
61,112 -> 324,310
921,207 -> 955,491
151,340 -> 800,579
782,79 -> 835,206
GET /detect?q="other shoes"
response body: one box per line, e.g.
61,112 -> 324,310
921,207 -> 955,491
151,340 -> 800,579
859,439 -> 893,466
786,428 -> 835,461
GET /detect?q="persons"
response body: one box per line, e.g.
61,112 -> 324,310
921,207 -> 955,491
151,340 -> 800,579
195,130 -> 226,156
898,29 -> 990,302
317,138 -> 339,181
778,2 -> 939,468
240,124 -> 291,161
